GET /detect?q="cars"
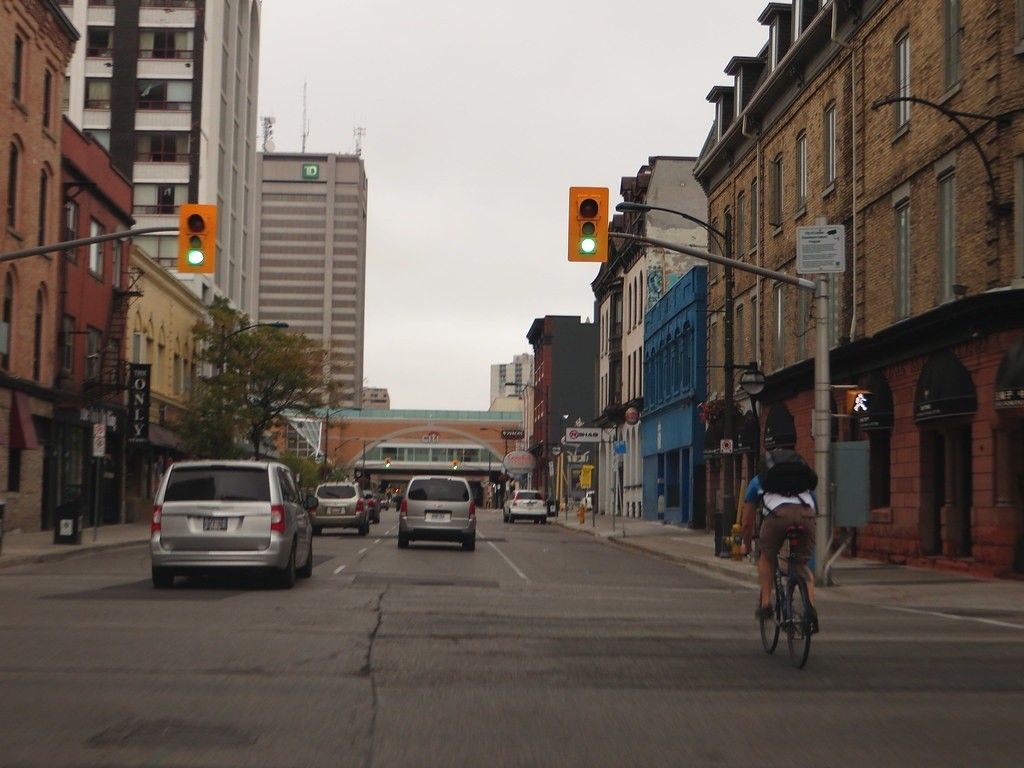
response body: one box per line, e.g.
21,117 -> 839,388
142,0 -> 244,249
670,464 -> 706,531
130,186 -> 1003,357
308,482 -> 370,535
503,490 -> 557,525
400,474 -> 477,551
581,491 -> 595,511
150,459 -> 312,590
366,497 -> 381,525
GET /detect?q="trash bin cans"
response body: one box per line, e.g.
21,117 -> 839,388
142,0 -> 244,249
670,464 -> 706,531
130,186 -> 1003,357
715,511 -> 737,556
54,505 -> 84,544
547,500 -> 560,516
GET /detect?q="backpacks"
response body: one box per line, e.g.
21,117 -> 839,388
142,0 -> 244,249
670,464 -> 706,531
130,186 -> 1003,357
755,448 -> 818,496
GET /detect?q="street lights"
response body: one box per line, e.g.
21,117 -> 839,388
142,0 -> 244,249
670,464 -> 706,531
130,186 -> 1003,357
215,322 -> 290,433
323,407 -> 361,482
333,437 -> 360,469
617,202 -> 735,557
504,382 -> 549,498
479,427 -> 507,509
718,362 -> 767,558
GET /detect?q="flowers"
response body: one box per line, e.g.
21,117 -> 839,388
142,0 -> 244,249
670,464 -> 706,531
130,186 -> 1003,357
699,398 -> 753,437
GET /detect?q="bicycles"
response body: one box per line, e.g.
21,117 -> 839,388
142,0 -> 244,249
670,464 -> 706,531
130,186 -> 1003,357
734,526 -> 817,669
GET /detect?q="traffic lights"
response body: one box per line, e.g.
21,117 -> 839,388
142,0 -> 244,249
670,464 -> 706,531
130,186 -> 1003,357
178,204 -> 218,274
453,460 -> 458,470
846,389 -> 873,415
385,457 -> 390,468
568,186 -> 609,262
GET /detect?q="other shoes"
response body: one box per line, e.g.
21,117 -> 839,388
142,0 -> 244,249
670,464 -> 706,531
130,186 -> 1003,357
808,608 -> 819,634
755,606 -> 772,617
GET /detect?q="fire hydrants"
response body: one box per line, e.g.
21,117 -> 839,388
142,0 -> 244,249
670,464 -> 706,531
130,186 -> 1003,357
576,503 -> 586,524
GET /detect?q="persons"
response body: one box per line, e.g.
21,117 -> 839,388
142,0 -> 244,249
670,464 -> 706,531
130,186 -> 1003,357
739,473 -> 819,637
